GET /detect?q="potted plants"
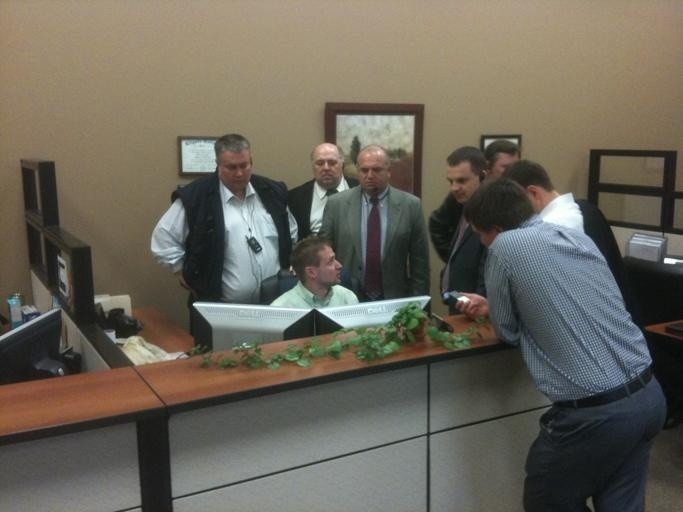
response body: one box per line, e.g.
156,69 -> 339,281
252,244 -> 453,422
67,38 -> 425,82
185,301 -> 491,371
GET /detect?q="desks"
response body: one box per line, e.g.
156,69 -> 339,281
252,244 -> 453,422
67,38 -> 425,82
102,302 -> 196,356
644,320 -> 682,429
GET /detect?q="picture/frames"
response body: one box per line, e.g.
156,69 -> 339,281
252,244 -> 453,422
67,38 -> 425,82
177,135 -> 217,175
324,101 -> 424,207
480,134 -> 521,150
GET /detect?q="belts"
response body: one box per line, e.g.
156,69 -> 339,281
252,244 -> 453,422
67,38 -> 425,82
553,367 -> 653,411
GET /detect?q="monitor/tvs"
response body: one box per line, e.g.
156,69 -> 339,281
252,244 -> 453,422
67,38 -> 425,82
191,300 -> 314,353
0,308 -> 69,384
315,294 -> 431,336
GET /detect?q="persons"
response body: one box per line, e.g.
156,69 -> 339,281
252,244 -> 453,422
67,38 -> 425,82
502,158 -> 633,304
316,144 -> 431,320
281,141 -> 357,240
454,176 -> 668,512
428,139 -> 521,260
267,237 -> 359,307
149,134 -> 299,354
438,147 -> 488,316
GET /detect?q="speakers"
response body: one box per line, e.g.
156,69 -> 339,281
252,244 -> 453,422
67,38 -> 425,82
63,353 -> 81,372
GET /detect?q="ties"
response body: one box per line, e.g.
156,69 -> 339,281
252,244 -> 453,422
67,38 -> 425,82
365,195 -> 383,300
